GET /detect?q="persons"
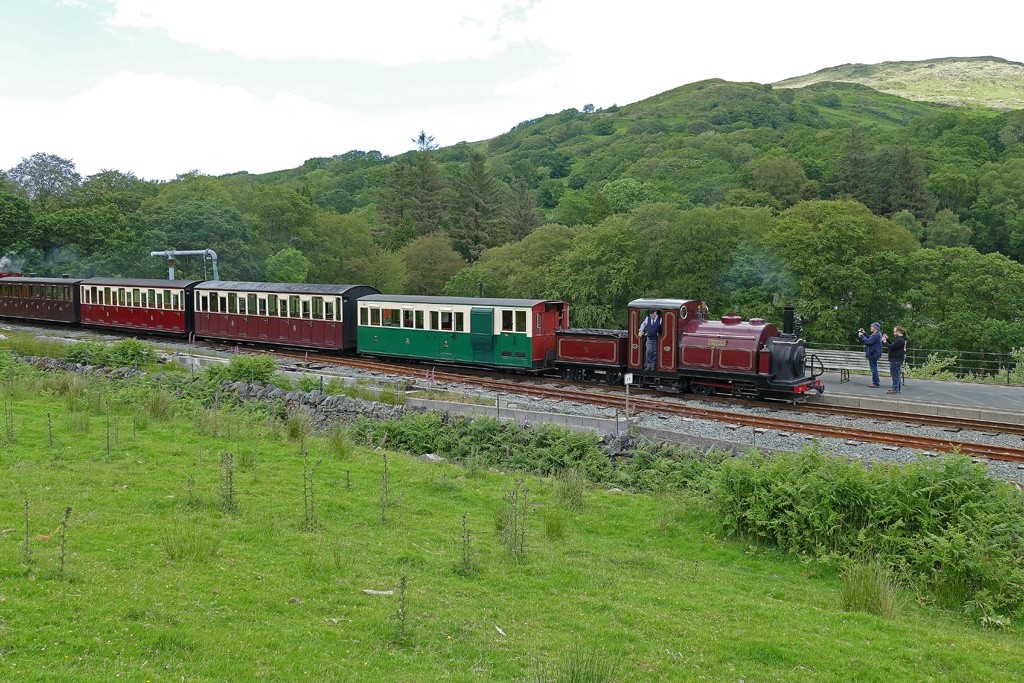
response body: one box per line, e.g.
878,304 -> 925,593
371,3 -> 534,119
858,322 -> 882,388
638,308 -> 663,370
882,326 -> 909,394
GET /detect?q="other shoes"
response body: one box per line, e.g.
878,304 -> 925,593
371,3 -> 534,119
867,384 -> 880,388
645,367 -> 655,371
886,389 -> 899,395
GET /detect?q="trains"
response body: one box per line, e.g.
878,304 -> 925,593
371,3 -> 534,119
0,271 -> 827,402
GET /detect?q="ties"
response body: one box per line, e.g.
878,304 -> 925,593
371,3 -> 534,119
653,319 -> 654,324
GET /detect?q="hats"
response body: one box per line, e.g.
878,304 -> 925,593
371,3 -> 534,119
870,322 -> 881,330
648,309 -> 657,313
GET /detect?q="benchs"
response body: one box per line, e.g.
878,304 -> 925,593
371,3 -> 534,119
805,348 -> 906,385
